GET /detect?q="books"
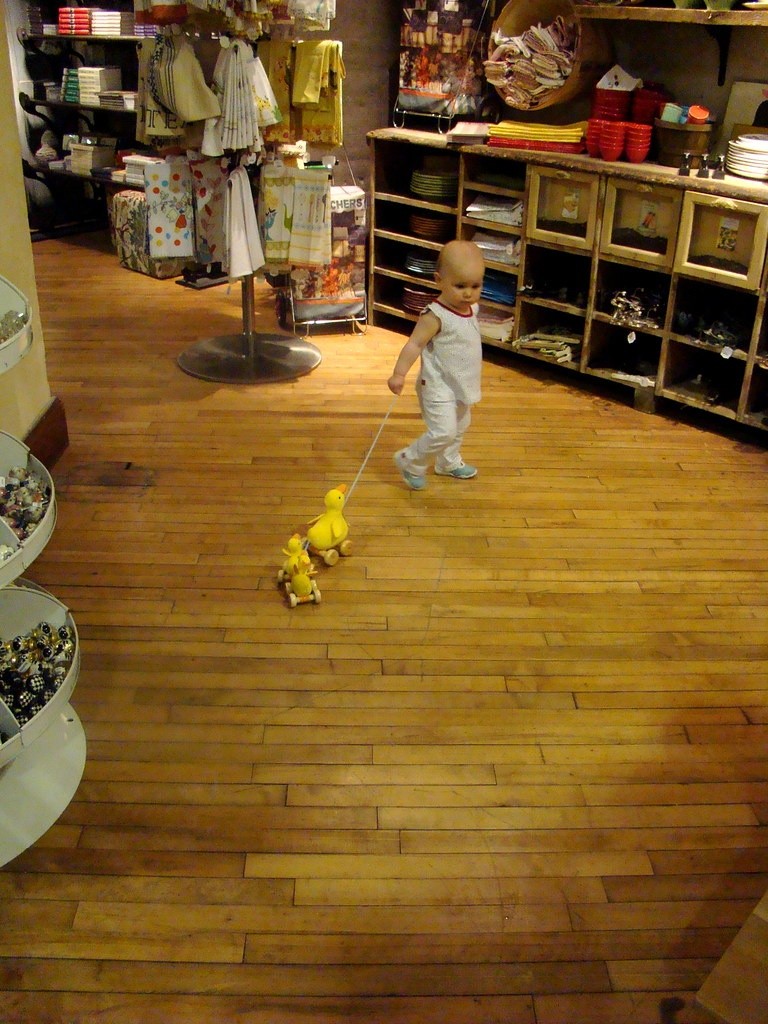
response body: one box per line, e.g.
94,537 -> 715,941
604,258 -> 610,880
25,5 -> 166,185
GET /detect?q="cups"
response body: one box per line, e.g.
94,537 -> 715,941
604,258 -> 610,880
586,85 -> 673,162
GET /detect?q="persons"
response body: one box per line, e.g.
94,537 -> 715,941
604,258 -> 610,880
388,239 -> 486,490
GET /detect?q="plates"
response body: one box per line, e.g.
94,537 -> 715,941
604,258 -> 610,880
726,133 -> 768,180
403,253 -> 439,276
410,213 -> 454,244
400,287 -> 442,313
407,168 -> 457,203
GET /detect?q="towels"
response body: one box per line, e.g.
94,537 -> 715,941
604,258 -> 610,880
134,0 -> 344,279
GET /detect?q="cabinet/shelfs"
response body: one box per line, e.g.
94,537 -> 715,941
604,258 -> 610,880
20,30 -> 148,191
365,125 -> 768,438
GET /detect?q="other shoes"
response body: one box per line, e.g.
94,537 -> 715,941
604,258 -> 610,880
435,461 -> 477,478
396,449 -> 425,490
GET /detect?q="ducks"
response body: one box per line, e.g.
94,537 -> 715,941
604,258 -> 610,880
281,482 -> 350,597
